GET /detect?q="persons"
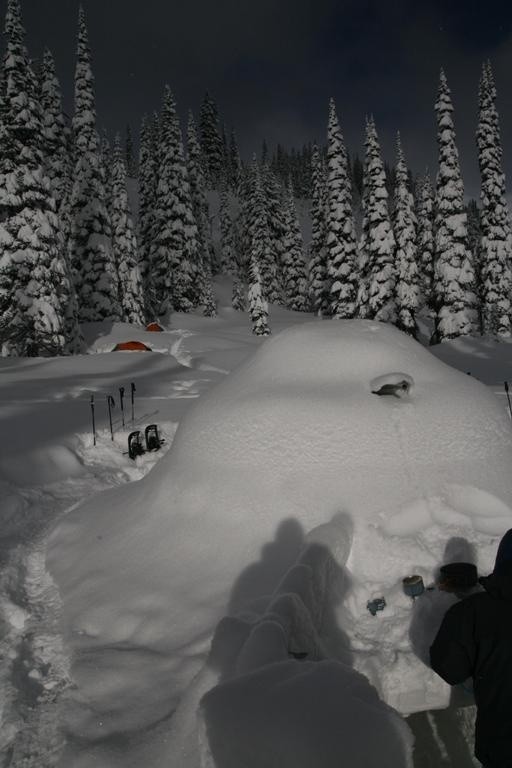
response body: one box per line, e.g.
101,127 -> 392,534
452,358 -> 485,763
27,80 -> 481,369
427,528 -> 512,767
